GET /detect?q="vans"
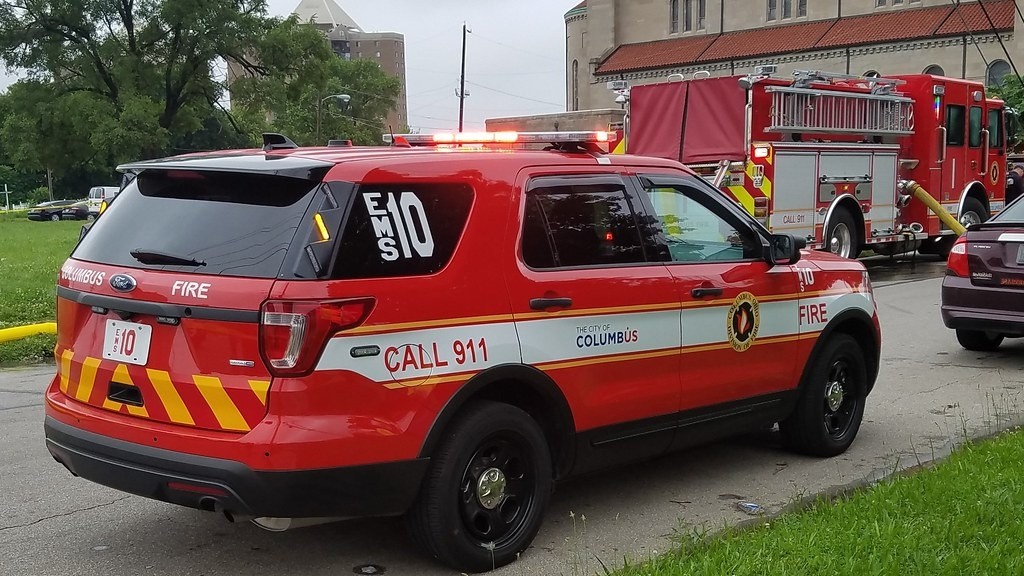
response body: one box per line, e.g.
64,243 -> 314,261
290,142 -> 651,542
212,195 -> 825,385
88,186 -> 121,218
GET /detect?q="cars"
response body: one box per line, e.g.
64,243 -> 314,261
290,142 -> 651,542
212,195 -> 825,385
61,200 -> 89,220
940,190 -> 1024,349
27,200 -> 75,221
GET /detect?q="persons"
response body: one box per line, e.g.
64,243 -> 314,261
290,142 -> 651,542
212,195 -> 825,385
1005,165 -> 1024,208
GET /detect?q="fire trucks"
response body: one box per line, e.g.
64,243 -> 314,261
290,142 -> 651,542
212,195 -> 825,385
606,63 -> 1020,264
45,128 -> 882,573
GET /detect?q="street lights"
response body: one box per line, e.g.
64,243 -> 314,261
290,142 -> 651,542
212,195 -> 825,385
317,93 -> 351,144
